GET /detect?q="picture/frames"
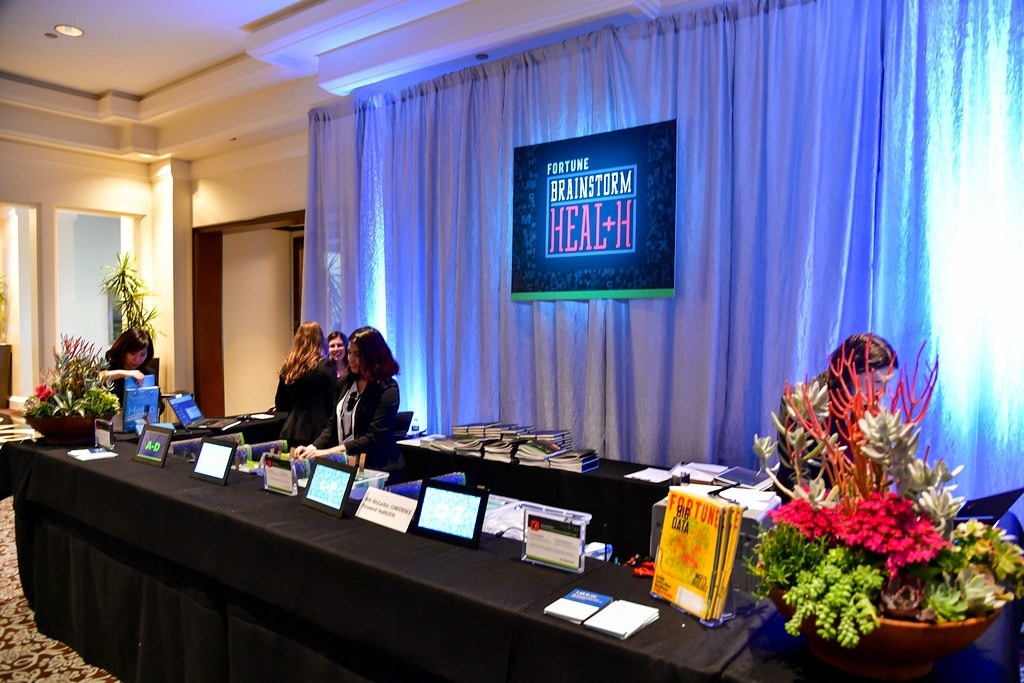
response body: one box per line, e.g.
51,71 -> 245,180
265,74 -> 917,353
132,424 -> 174,468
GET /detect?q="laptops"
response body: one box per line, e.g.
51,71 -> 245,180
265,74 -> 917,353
394,411 -> 415,437
166,393 -> 237,429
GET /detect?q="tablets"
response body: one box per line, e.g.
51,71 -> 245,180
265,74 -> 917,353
409,479 -> 490,550
188,437 -> 239,487
301,455 -> 357,519
132,424 -> 174,468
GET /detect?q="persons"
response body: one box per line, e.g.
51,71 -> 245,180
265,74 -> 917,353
326,331 -> 349,381
772,333 -> 899,505
294,327 -> 401,486
92,329 -> 166,416
275,320 -> 337,447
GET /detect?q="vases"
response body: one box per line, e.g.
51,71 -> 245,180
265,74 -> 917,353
765,587 -> 1005,683
24,413 -> 115,446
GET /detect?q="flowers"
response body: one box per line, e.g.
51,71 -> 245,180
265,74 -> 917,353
19,334 -> 122,418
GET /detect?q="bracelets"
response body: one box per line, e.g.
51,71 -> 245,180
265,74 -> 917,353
307,444 -> 316,450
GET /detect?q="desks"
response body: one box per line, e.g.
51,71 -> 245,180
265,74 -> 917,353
0,411 -> 1024,683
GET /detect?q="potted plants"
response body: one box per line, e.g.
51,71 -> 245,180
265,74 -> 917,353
738,332 -> 1024,649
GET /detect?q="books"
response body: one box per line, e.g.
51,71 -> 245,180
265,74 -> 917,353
122,374 -> 159,433
543,588 -> 660,640
652,486 -> 742,620
429,422 -> 599,473
